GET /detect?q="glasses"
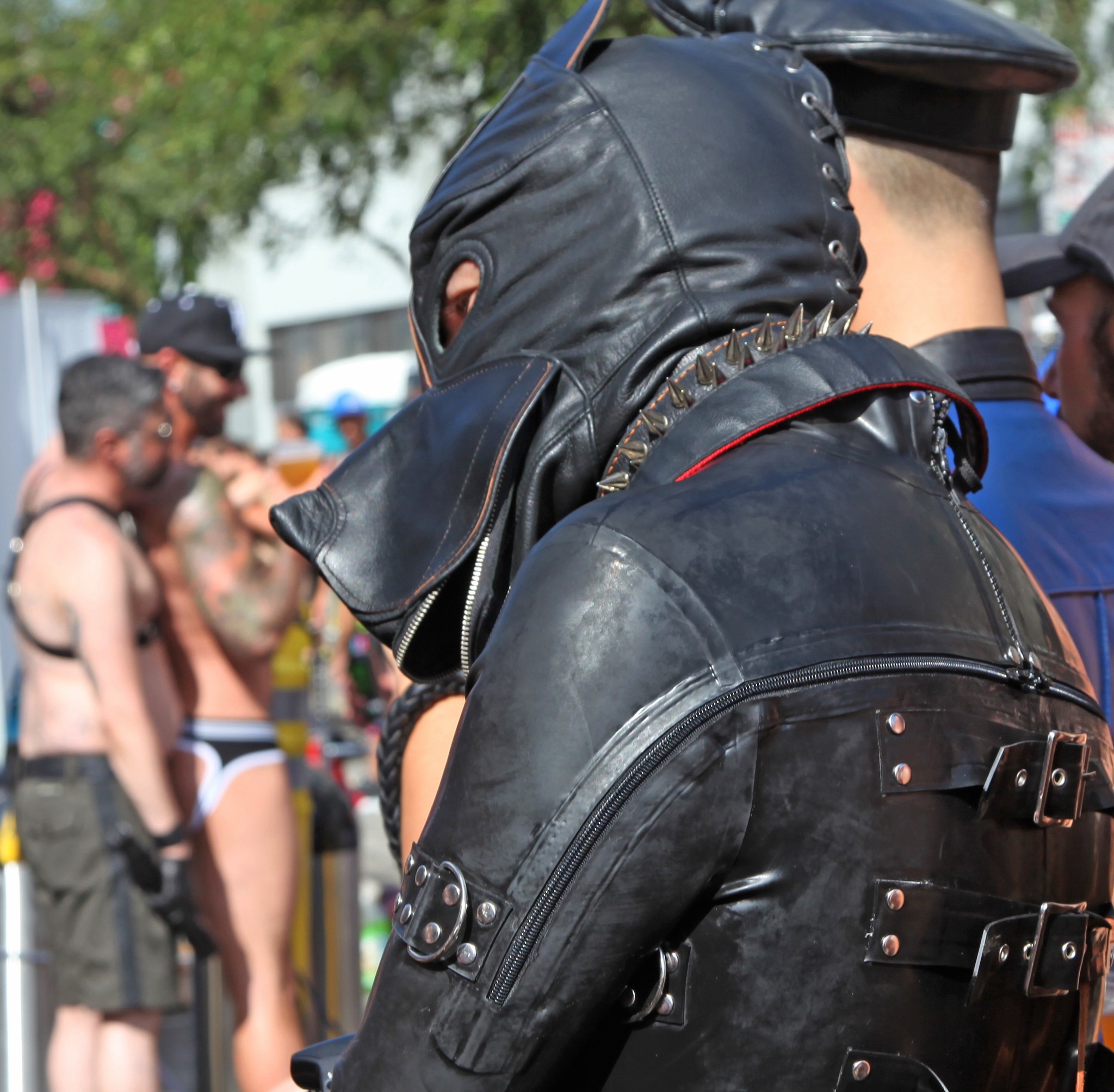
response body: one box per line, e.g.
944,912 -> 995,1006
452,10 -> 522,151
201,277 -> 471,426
177,348 -> 245,380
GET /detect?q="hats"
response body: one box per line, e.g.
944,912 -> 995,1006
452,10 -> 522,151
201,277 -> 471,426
649,1 -> 1081,152
998,167 -> 1114,299
330,393 -> 368,420
135,295 -> 271,363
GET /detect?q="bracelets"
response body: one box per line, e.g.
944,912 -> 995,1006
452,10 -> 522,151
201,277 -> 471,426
142,815 -> 188,850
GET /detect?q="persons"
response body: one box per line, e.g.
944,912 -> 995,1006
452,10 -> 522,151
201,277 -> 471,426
0,282 -> 429,1092
283,0 -> 1114,1092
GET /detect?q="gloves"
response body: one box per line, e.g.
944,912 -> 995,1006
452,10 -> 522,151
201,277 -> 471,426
142,852 -> 199,929
